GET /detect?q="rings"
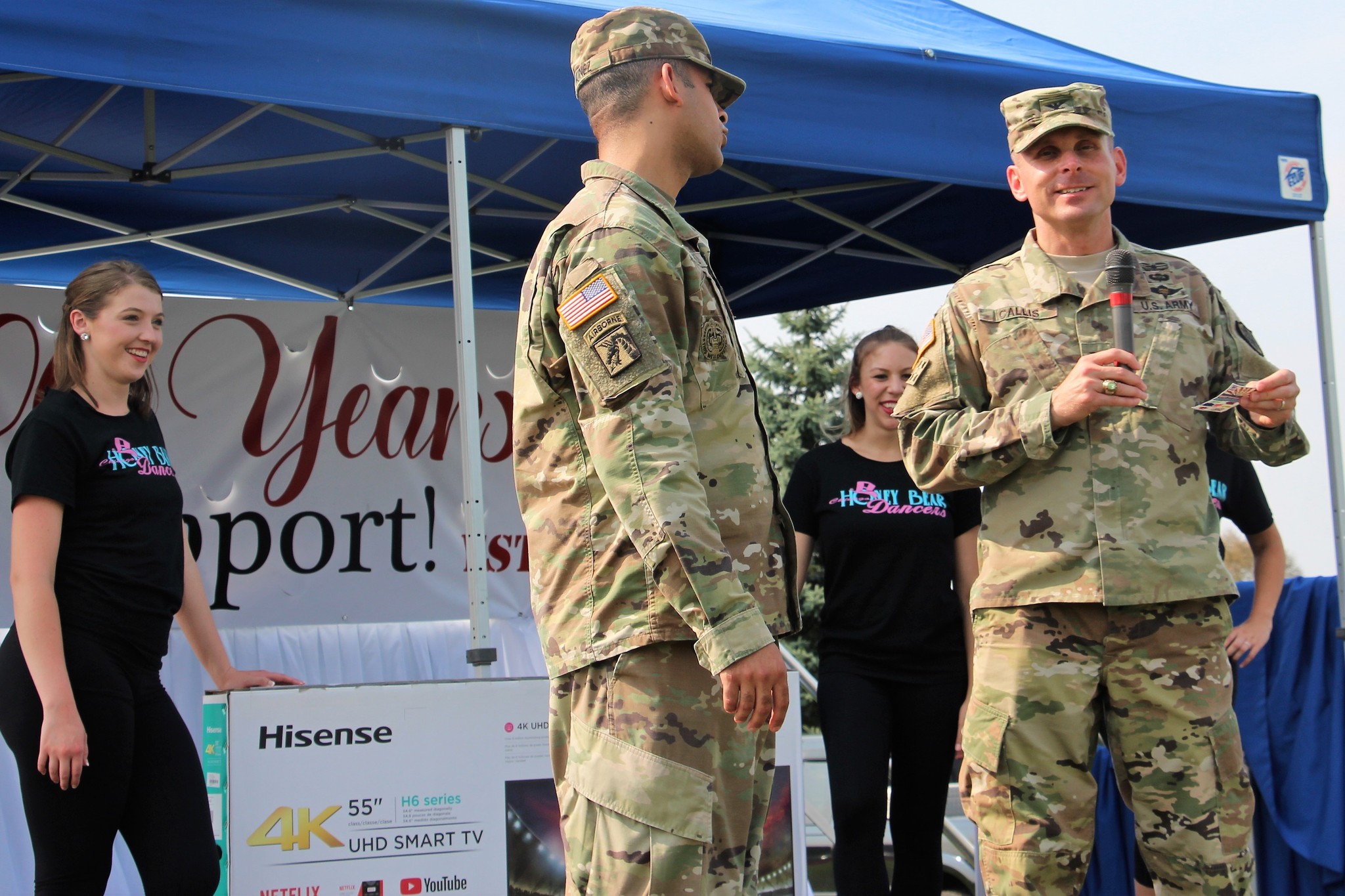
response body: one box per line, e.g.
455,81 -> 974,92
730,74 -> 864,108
1101,380 -> 1118,396
1277,400 -> 1285,411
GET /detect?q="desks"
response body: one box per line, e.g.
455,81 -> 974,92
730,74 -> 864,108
202,667 -> 805,896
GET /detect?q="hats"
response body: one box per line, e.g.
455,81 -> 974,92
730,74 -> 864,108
569,8 -> 746,110
999,81 -> 1114,154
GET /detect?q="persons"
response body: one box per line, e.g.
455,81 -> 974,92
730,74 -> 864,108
888,82 -> 1311,896
0,259 -> 306,896
510,6 -> 805,896
777,326 -> 983,896
1122,430 -> 1286,896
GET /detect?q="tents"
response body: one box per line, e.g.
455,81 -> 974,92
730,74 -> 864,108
0,0 -> 1344,675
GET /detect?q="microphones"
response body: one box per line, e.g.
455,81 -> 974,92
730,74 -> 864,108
1104,249 -> 1136,384
